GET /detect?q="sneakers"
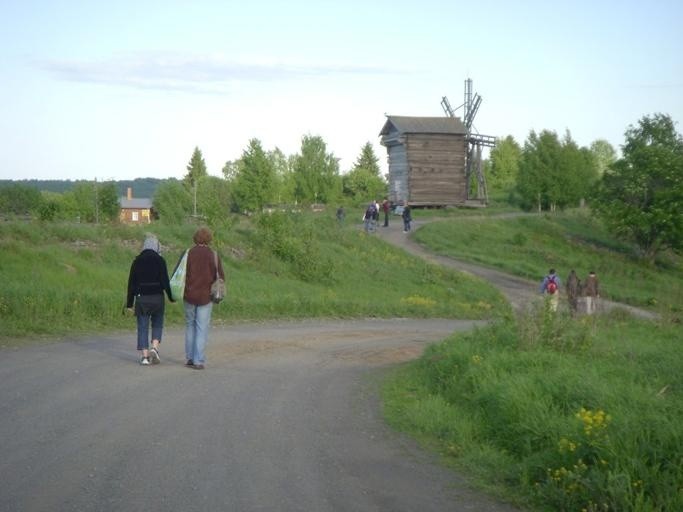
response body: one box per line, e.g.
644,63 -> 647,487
141,356 -> 152,365
186,359 -> 205,370
149,347 -> 161,364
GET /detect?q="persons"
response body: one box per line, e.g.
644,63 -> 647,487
539,268 -> 562,312
361,196 -> 390,233
402,200 -> 411,234
582,270 -> 602,316
125,235 -> 176,366
335,205 -> 346,222
172,226 -> 225,369
566,270 -> 581,319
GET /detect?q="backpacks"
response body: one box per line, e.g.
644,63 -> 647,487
546,275 -> 557,294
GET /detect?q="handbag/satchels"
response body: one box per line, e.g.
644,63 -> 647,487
210,251 -> 225,303
169,247 -> 191,301
